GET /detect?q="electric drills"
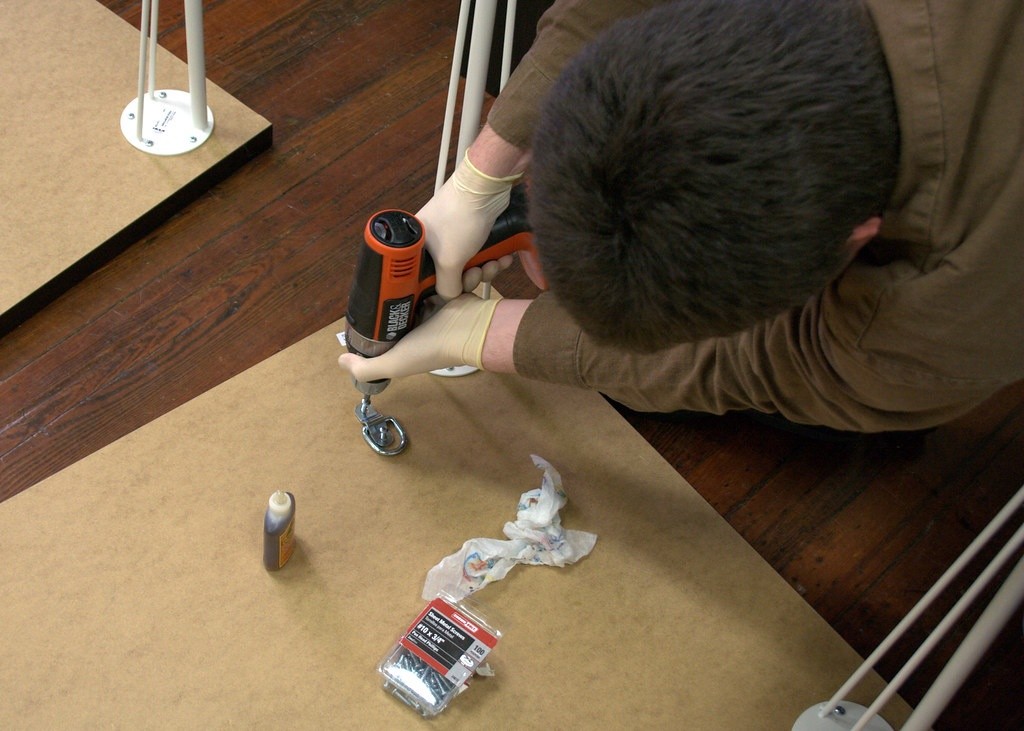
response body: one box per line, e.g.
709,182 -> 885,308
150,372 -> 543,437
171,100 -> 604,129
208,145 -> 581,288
344,183 -> 551,404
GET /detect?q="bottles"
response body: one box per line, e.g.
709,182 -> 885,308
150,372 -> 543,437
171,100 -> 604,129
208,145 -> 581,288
264,488 -> 296,572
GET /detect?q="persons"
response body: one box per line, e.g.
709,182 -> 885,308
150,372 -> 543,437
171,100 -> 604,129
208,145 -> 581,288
336,0 -> 1024,434
